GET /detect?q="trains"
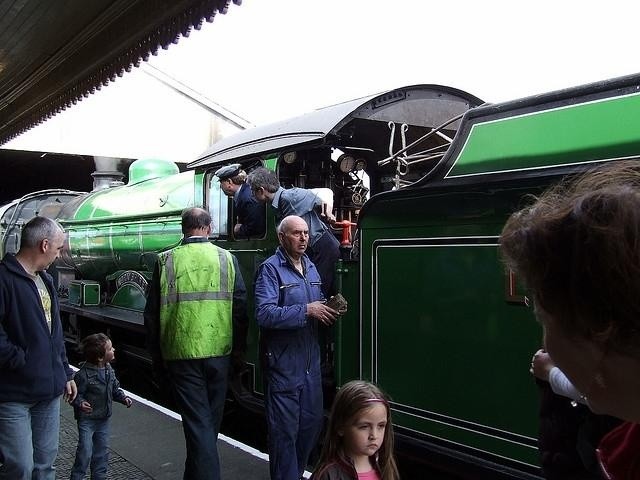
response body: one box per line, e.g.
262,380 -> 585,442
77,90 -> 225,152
0,72 -> 640,478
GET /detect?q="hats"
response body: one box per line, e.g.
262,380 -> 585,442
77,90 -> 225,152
214,163 -> 242,181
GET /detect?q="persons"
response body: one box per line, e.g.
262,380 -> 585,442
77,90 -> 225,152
251,212 -> 350,480
307,378 -> 404,479
245,165 -> 340,368
527,342 -> 626,480
142,206 -> 250,480
502,157 -> 638,479
71,331 -> 133,479
213,162 -> 267,241
0,214 -> 78,480
